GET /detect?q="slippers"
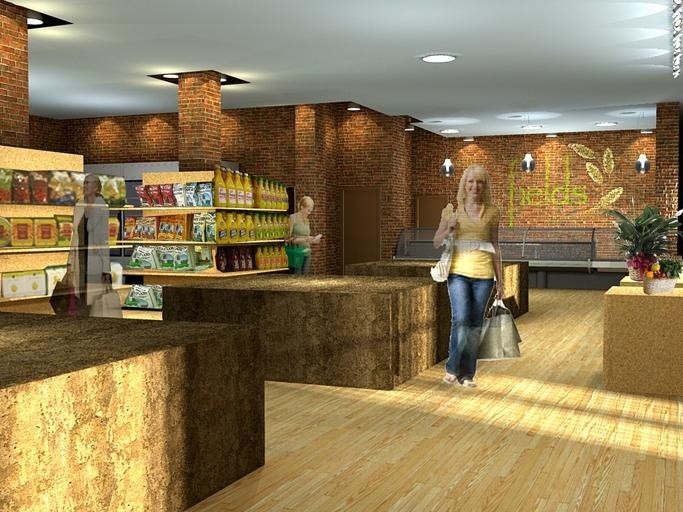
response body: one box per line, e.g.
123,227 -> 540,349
443,374 -> 457,384
463,380 -> 477,388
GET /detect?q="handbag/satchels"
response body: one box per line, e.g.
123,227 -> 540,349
50,273 -> 69,315
475,297 -> 523,363
69,273 -> 78,317
91,278 -> 124,319
284,238 -> 309,269
430,233 -> 454,284
